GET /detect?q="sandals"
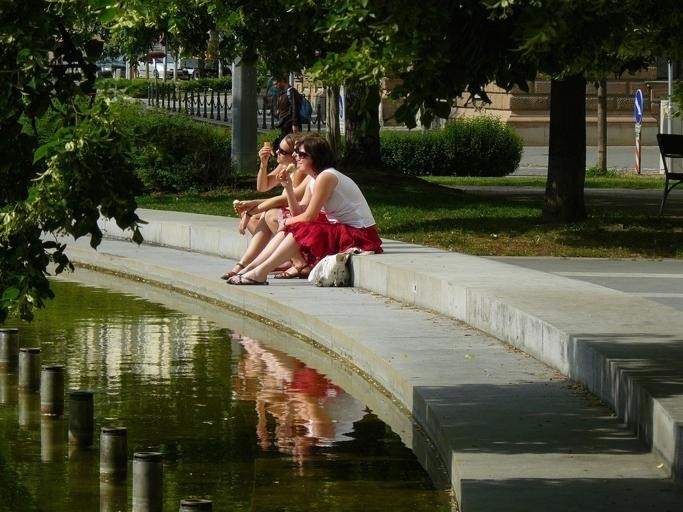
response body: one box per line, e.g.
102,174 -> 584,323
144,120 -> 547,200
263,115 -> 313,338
273,263 -> 311,279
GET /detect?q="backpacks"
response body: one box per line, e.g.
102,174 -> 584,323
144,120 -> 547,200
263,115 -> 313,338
286,86 -> 312,124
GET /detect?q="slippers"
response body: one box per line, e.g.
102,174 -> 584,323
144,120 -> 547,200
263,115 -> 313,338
221,263 -> 269,285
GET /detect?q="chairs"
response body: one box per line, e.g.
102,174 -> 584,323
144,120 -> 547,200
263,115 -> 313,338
657,133 -> 683,214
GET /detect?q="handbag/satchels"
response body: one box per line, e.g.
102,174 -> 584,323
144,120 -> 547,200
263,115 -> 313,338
308,247 -> 375,288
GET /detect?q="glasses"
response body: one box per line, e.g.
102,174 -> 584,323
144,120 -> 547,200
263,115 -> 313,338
278,145 -> 290,155
294,148 -> 310,159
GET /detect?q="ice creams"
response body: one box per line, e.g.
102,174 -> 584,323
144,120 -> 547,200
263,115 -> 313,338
264,142 -> 275,156
286,164 -> 296,173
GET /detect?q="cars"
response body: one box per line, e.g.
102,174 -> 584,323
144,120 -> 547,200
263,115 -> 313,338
96,52 -> 231,80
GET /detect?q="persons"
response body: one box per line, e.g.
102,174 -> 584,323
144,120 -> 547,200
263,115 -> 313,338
218,132 -> 384,286
269,75 -> 302,159
228,335 -> 369,479
